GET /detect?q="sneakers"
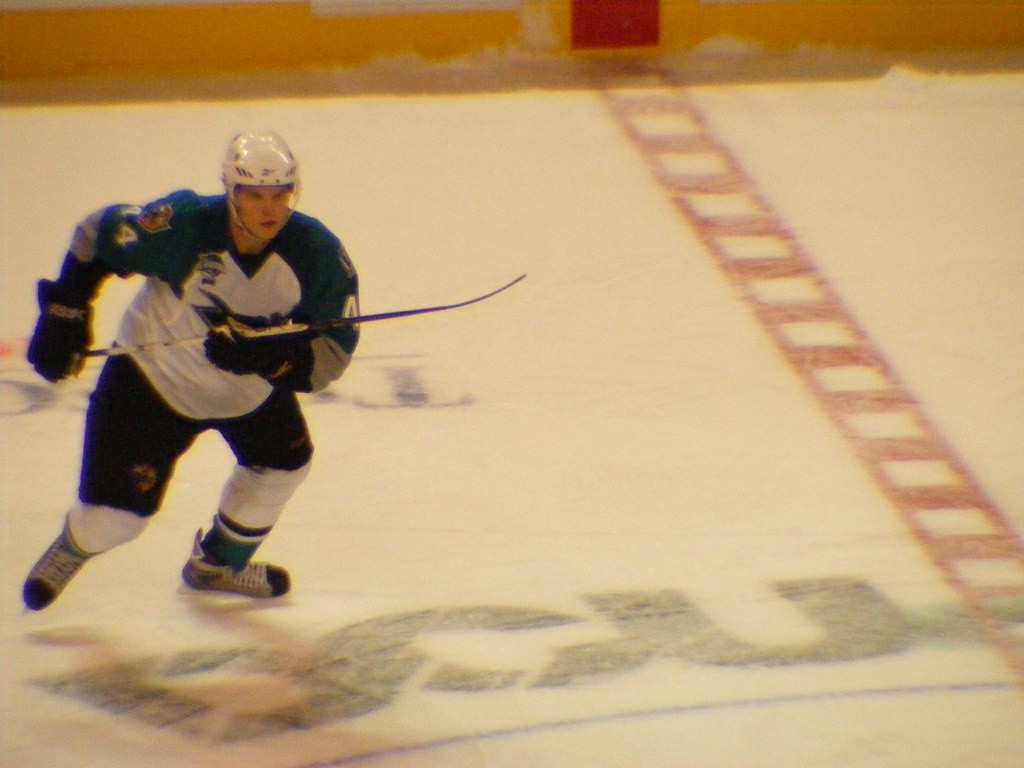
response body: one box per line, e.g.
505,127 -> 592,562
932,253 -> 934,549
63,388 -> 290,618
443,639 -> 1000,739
176,527 -> 291,598
22,512 -> 104,614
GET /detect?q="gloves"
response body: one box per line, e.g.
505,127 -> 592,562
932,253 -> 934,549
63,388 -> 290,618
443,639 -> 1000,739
27,280 -> 92,383
204,314 -> 305,385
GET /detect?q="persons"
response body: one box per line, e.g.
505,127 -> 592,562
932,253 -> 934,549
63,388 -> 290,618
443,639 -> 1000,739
20,132 -> 361,611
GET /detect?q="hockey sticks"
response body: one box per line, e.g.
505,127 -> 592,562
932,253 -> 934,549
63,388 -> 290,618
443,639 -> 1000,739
80,267 -> 534,361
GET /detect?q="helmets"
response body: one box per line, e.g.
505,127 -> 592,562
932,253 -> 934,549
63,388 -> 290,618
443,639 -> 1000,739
221,131 -> 301,243
